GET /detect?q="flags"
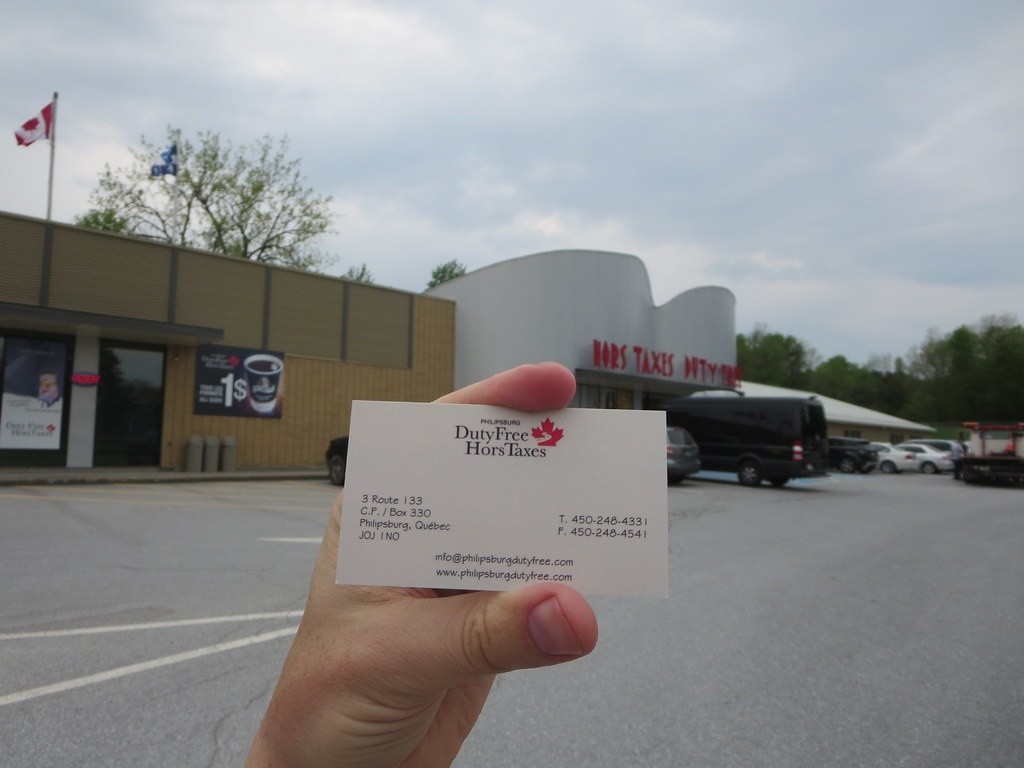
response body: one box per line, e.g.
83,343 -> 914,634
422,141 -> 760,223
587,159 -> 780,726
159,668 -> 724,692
148,143 -> 177,176
13,103 -> 52,147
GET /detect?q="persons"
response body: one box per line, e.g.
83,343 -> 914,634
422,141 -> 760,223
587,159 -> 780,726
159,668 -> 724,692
959,439 -> 968,480
951,439 -> 965,480
244,360 -> 598,768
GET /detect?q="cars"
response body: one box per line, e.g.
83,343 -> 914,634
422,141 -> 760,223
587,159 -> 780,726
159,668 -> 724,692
665,426 -> 702,487
870,441 -> 918,475
326,434 -> 349,486
905,439 -> 966,459
830,437 -> 878,474
894,443 -> 956,474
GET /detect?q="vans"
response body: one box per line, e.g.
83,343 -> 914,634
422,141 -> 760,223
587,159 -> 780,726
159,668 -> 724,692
661,389 -> 831,488
